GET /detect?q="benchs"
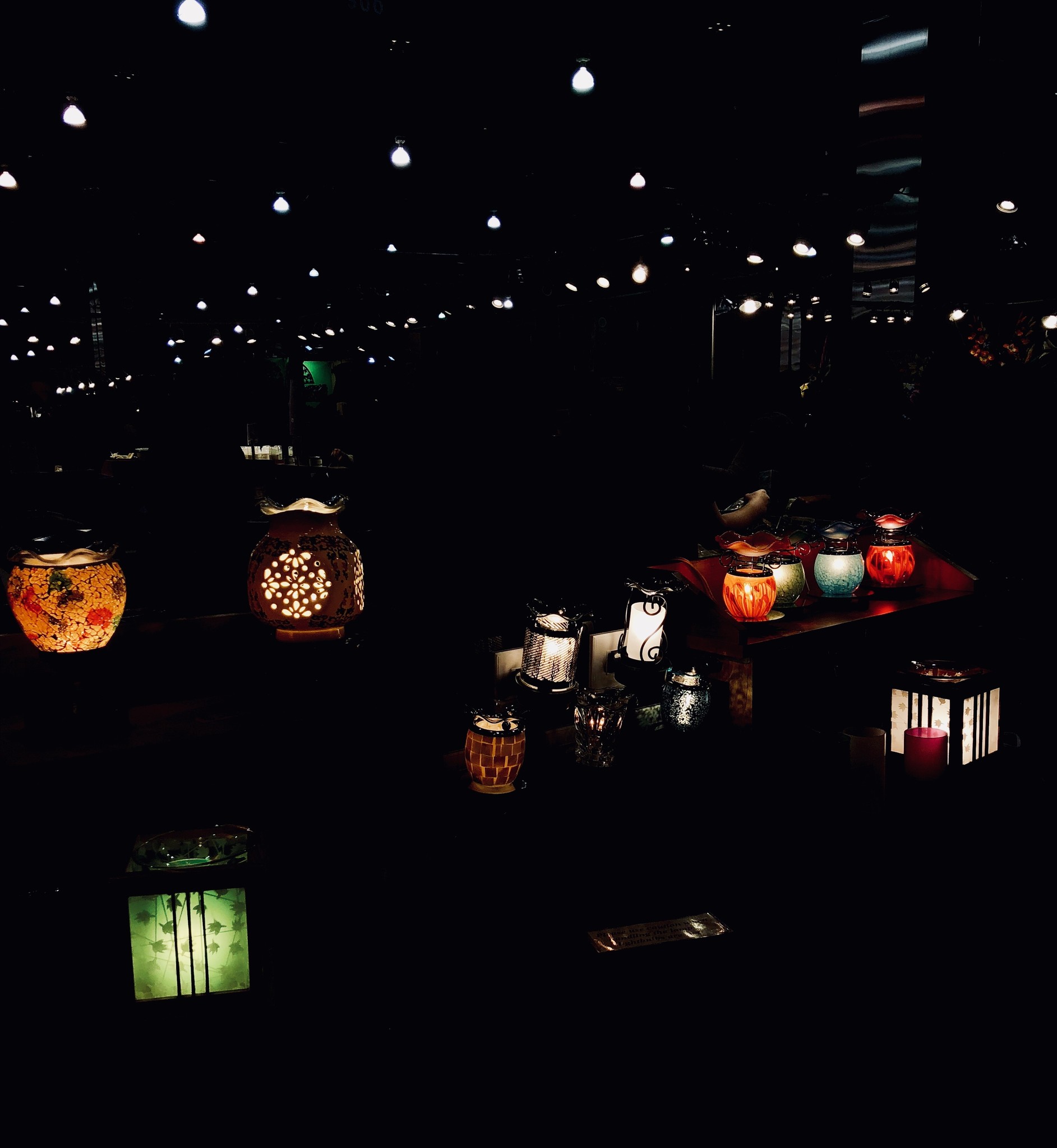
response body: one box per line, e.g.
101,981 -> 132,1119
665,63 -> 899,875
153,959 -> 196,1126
648,531 -> 1001,713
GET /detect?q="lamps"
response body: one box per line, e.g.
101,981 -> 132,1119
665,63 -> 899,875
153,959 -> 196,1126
467,491 -> 999,800
248,492 -> 365,642
5,539 -> 129,655
124,826 -> 256,1001
